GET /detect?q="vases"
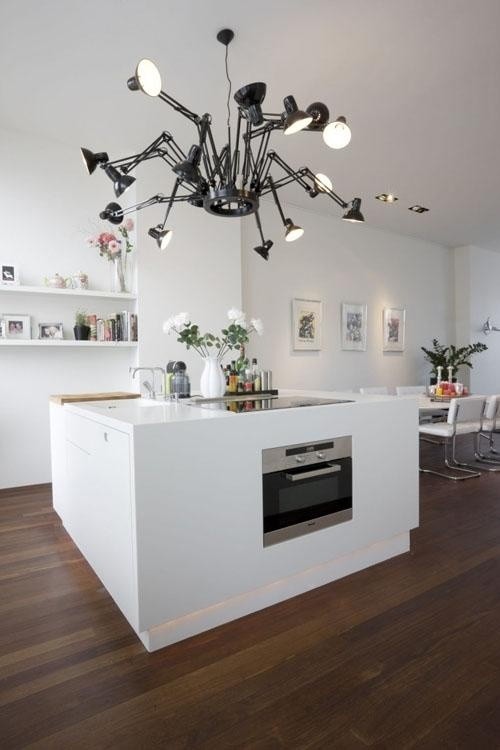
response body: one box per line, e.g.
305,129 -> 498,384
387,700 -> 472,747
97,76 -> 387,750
113,258 -> 124,292
200,357 -> 226,399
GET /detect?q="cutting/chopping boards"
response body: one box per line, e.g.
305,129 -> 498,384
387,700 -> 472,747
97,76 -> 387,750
49,392 -> 143,405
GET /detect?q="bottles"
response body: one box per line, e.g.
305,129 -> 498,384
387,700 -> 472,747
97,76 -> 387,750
171,363 -> 189,398
230,400 -> 262,413
238,374 -> 245,393
251,358 -> 262,392
225,360 -> 235,391
166,362 -> 176,396
244,369 -> 255,392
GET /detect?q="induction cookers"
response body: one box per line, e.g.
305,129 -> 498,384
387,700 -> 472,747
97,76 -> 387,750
188,395 -> 355,413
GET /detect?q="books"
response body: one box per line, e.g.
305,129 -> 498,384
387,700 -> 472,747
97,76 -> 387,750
88,309 -> 137,341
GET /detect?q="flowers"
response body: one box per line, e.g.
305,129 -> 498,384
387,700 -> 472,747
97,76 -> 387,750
163,306 -> 264,357
85,218 -> 135,264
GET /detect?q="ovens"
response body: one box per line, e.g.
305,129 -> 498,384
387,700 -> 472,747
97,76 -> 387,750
262,433 -> 352,550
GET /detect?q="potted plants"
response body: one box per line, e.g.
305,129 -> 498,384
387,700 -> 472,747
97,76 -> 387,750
73,307 -> 90,340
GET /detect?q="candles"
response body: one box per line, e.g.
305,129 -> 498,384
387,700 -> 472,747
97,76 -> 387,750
437,365 -> 442,384
449,364 -> 452,383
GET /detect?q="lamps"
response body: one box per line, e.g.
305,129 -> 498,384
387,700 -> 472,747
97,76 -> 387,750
79,30 -> 365,262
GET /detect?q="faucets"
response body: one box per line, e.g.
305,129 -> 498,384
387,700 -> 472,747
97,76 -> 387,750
132,367 -> 155,388
153,366 -> 167,396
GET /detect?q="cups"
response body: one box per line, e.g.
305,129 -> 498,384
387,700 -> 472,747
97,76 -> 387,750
261,400 -> 272,410
260,370 -> 272,391
439,384 -> 449,395
453,383 -> 464,396
71,269 -> 88,291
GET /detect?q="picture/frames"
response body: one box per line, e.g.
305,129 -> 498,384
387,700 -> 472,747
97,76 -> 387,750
1,313 -> 32,339
1,263 -> 19,284
383,306 -> 407,352
38,322 -> 63,339
341,303 -> 368,352
291,297 -> 324,351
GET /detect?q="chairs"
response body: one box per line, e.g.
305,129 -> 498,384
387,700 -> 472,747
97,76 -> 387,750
360,385 -> 500,481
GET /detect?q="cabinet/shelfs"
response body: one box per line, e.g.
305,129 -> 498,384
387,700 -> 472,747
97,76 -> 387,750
0,284 -> 137,347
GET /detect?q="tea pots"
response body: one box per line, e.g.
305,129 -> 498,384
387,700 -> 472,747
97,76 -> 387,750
44,272 -> 73,290
